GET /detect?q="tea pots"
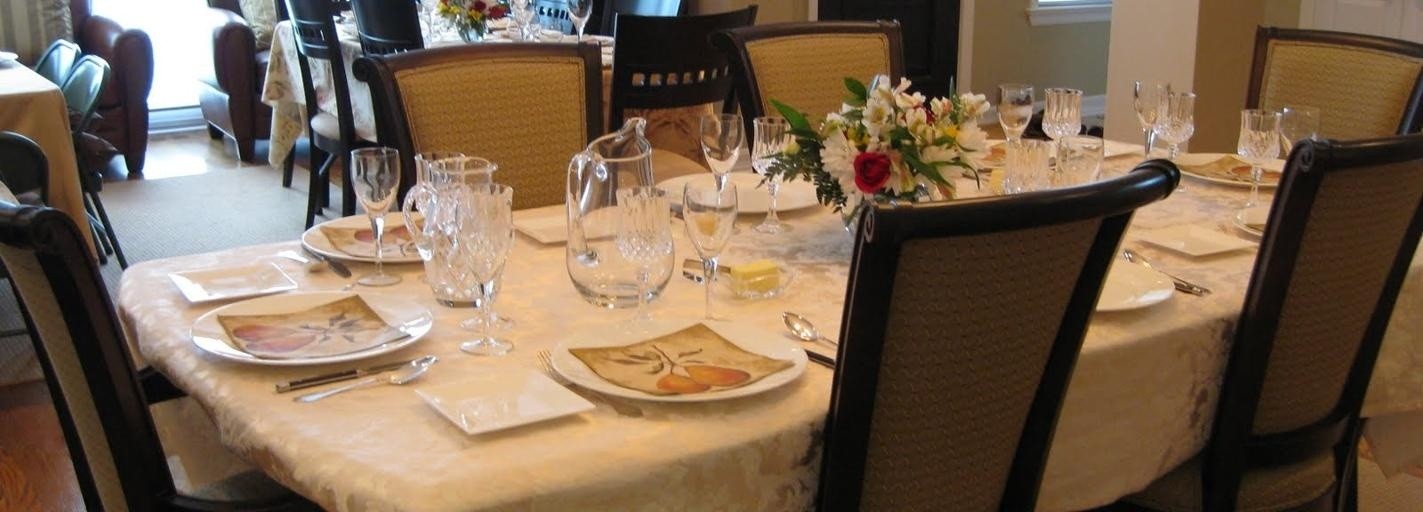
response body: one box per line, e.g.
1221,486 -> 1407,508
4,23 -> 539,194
403,158 -> 498,305
563,116 -> 674,311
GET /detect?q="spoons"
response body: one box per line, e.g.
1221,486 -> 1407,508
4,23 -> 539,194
298,357 -> 438,402
783,307 -> 843,368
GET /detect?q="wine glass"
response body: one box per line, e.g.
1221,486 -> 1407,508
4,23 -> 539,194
994,79 -> 1321,222
685,114 -> 740,236
349,145 -> 400,288
419,0 -> 598,52
746,119 -> 801,236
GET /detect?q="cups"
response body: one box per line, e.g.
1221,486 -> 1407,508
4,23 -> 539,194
413,150 -> 464,210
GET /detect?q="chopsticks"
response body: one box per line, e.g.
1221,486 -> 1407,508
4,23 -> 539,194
275,357 -> 414,394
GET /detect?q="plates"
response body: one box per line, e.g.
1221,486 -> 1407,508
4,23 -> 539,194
0,50 -> 18,69
301,213 -> 437,263
169,266 -> 301,307
190,289 -> 435,370
1090,223 -> 1268,316
551,317 -> 807,406
653,171 -> 822,210
